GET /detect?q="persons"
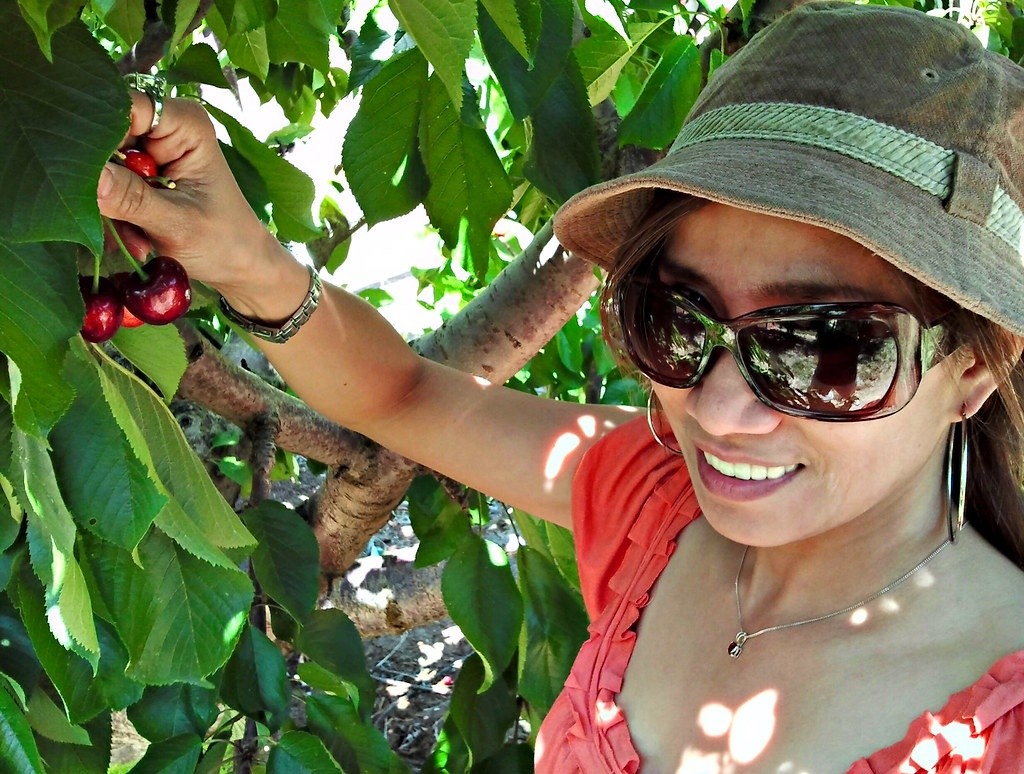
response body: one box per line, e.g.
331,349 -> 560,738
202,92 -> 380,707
96,5 -> 1024,774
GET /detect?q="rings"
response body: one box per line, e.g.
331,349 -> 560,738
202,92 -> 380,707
127,72 -> 166,134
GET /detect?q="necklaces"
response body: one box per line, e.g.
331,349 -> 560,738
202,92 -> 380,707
726,521 -> 970,659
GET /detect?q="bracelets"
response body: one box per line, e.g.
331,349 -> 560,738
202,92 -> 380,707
220,261 -> 323,344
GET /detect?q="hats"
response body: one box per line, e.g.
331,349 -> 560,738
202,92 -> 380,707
552,0 -> 1024,341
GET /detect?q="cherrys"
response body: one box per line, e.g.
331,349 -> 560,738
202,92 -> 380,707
78,147 -> 192,343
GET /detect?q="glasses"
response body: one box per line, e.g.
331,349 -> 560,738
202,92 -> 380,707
617,277 -> 989,423
